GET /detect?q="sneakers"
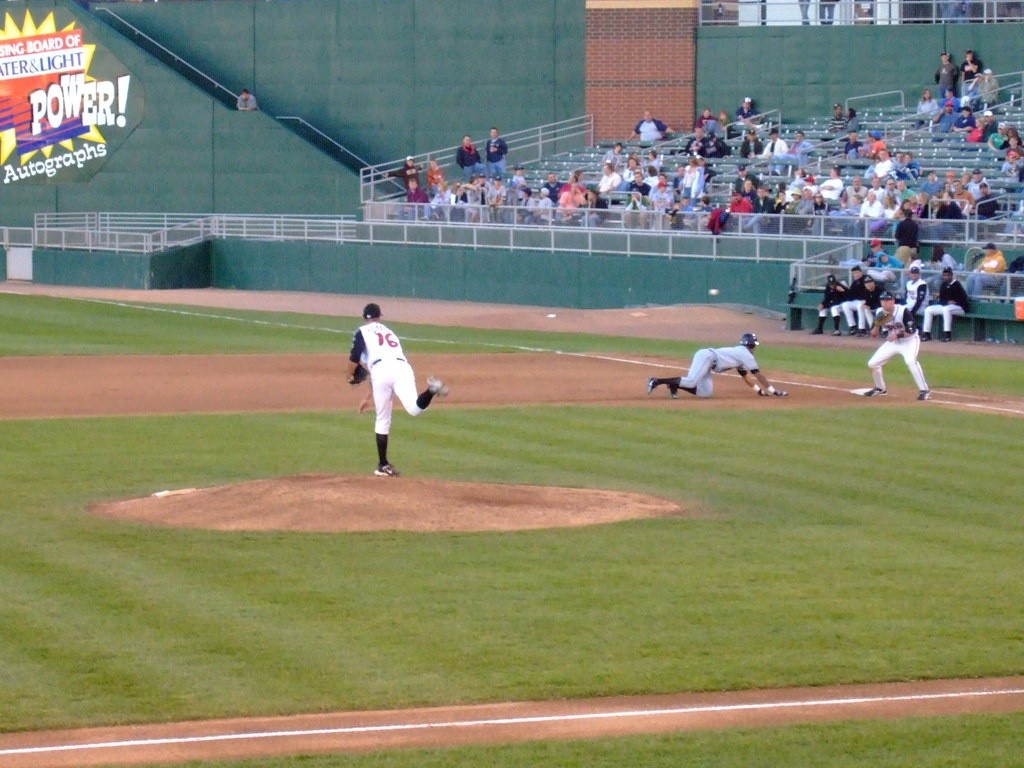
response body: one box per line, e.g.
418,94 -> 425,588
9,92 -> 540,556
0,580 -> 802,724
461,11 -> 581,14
864,386 -> 888,396
918,390 -> 931,400
668,383 -> 678,399
374,463 -> 400,477
647,376 -> 658,393
426,375 -> 449,396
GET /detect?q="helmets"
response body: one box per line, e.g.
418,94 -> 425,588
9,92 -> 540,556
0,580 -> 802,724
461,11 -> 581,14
879,290 -> 895,299
740,333 -> 760,345
911,266 -> 919,274
864,275 -> 873,282
942,266 -> 953,274
828,274 -> 836,283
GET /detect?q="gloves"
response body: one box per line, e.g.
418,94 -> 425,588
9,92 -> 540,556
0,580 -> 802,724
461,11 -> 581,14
768,385 -> 791,396
753,384 -> 769,396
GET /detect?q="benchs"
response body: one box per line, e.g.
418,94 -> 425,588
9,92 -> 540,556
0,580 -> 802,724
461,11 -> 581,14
387,87 -> 1024,237
782,302 -> 1024,344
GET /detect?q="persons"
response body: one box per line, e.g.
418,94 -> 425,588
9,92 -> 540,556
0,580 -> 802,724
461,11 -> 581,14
813,266 -> 969,342
875,253 -> 905,269
350,302 -> 449,477
959,49 -> 983,99
798,0 -> 809,25
820,0 -> 840,25
862,291 -> 932,400
384,68 -> 1024,241
925,246 -> 957,268
1000,255 -> 1024,301
858,240 -> 888,266
966,243 -> 1007,299
646,333 -> 789,399
935,52 -> 959,99
896,209 -> 918,266
236,89 -> 259,112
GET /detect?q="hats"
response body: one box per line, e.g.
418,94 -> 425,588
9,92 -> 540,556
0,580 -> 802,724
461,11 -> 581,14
869,240 -> 882,246
363,303 -> 383,318
982,243 -> 996,249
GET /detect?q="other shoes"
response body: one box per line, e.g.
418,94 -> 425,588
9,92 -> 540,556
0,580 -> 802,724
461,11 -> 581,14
940,335 -> 952,342
849,326 -> 859,335
833,329 -> 841,336
812,329 -> 823,334
856,329 -> 866,337
920,333 -> 931,341
881,330 -> 888,338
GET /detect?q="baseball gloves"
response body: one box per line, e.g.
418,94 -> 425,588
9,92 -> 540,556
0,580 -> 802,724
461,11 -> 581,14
874,310 -> 895,327
348,364 -> 369,384
